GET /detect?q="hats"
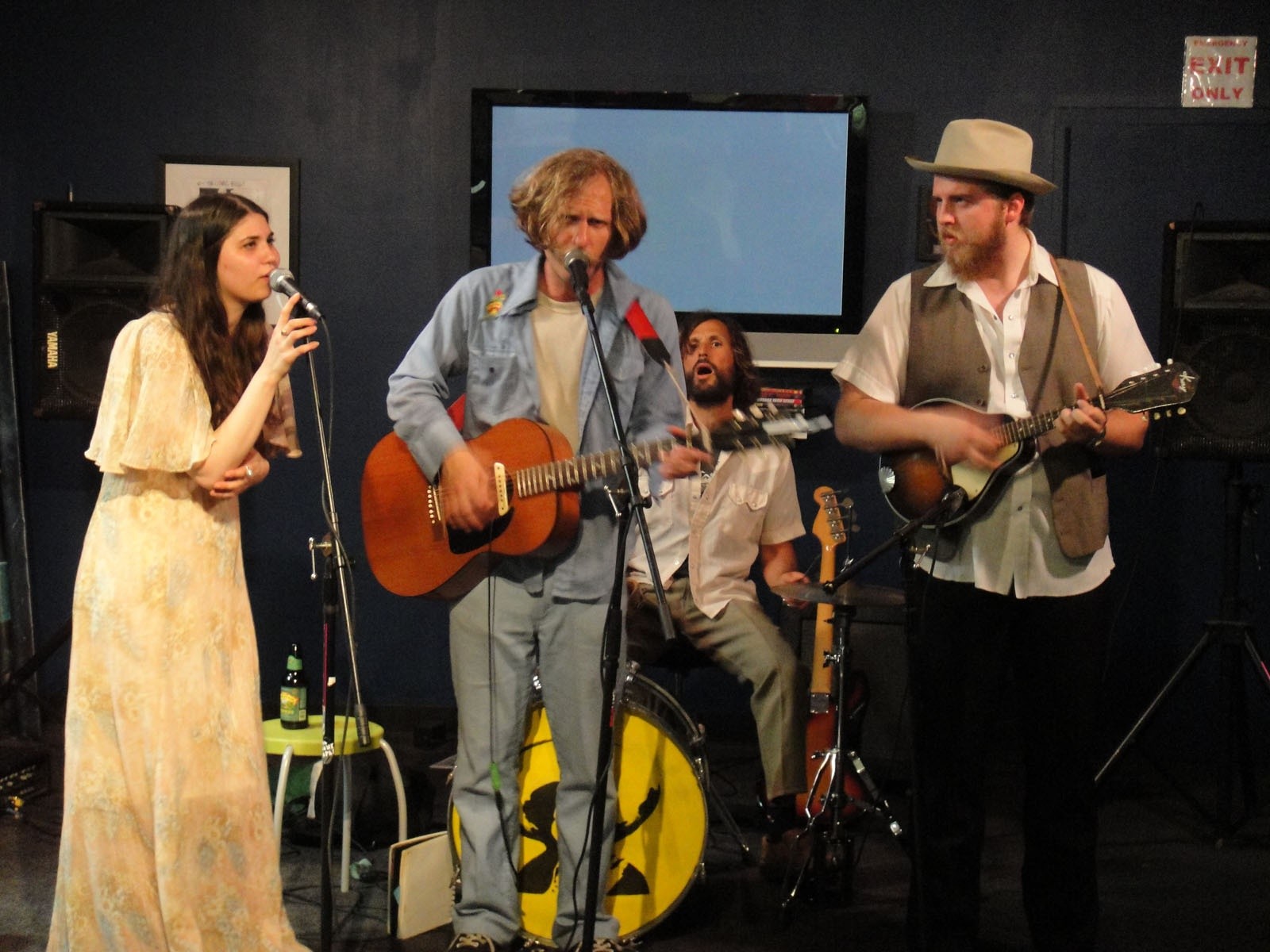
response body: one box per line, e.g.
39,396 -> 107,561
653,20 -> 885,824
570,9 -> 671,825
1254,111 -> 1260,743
904,118 -> 1058,196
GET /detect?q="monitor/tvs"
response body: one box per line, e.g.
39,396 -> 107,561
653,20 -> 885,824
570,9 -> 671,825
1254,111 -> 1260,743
470,86 -> 867,336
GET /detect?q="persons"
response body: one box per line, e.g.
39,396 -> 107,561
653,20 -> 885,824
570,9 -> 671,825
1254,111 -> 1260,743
46,192 -> 319,952
625,307 -> 837,890
386,149 -> 710,952
833,120 -> 1154,952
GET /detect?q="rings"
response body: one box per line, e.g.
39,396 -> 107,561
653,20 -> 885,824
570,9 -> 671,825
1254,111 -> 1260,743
246,465 -> 252,478
281,329 -> 289,337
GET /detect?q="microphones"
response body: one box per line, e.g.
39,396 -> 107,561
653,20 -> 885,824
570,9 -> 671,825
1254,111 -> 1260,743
563,249 -> 591,303
268,268 -> 326,323
935,485 -> 961,529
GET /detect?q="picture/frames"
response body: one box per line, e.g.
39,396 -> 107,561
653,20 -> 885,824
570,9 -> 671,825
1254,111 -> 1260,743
156,151 -> 299,291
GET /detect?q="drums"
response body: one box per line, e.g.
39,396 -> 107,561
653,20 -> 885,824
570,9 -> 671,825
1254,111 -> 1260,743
446,664 -> 709,948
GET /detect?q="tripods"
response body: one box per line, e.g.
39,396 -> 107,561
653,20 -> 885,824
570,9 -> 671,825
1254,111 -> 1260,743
768,606 -> 913,936
1095,459 -> 1270,851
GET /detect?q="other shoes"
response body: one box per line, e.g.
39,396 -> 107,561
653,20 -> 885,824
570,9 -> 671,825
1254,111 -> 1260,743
758,825 -> 832,874
448,930 -> 520,952
572,935 -> 622,952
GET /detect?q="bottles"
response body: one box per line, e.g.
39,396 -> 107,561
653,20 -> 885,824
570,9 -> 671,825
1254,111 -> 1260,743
280,642 -> 308,730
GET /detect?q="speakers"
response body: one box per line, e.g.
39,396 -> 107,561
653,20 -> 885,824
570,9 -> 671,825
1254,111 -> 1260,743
1158,219 -> 1269,460
25,201 -> 182,481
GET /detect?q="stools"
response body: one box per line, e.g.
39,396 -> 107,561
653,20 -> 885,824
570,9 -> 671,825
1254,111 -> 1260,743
260,714 -> 407,934
647,640 -> 757,870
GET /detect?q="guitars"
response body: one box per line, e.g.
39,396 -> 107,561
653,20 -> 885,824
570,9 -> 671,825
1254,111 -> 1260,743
879,357 -> 1195,531
756,486 -> 876,879
362,402 -> 833,600
